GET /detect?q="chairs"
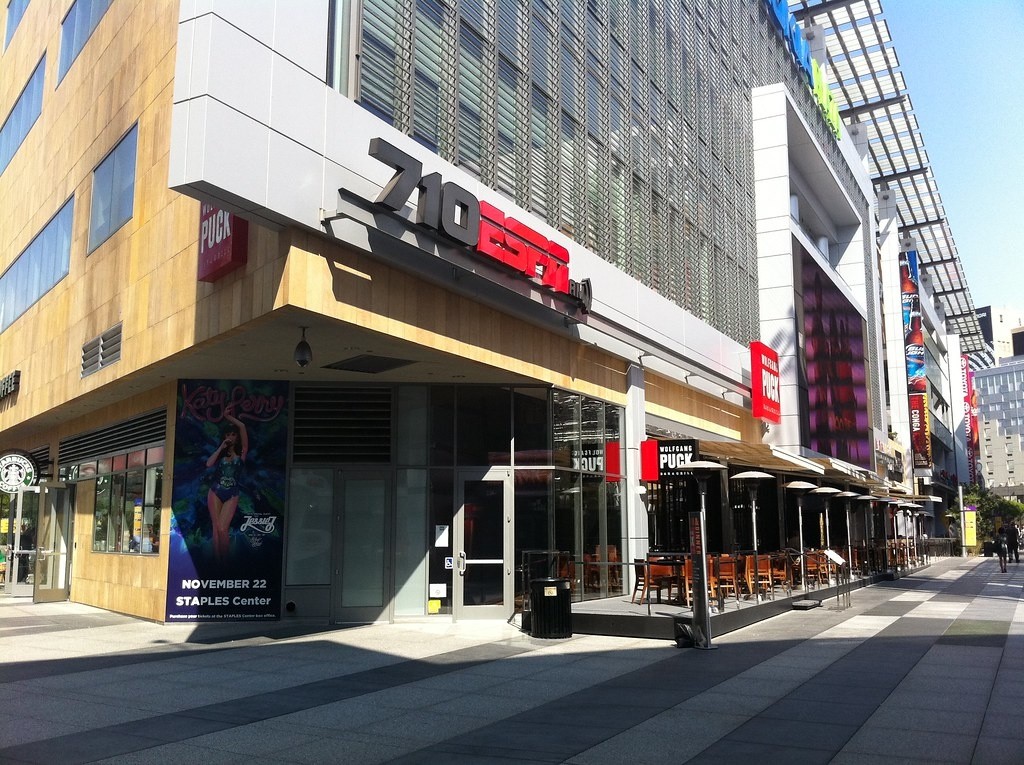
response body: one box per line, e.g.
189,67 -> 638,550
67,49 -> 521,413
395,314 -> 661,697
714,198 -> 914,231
562,544 -> 917,609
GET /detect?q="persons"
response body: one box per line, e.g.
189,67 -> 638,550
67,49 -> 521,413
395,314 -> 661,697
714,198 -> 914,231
992,526 -> 1007,575
205,404 -> 254,583
1014,523 -> 1024,552
144,524 -> 159,554
1008,522 -> 1020,562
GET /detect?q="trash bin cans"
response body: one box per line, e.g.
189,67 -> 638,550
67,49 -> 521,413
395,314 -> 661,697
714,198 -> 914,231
529,576 -> 574,639
